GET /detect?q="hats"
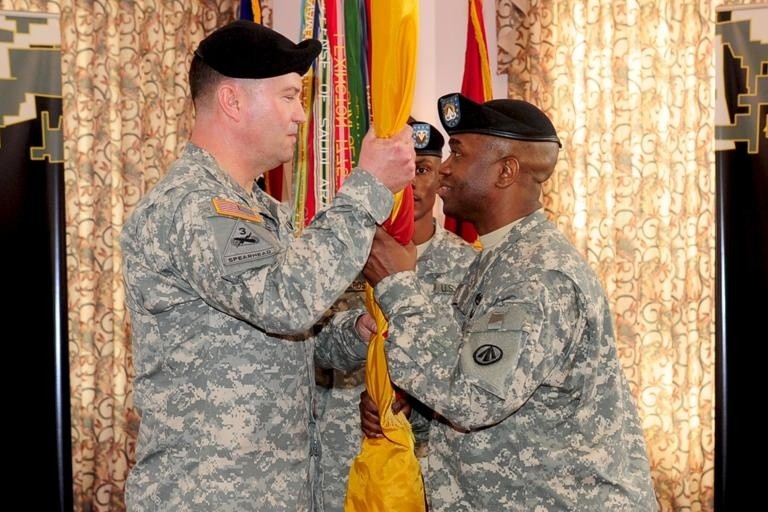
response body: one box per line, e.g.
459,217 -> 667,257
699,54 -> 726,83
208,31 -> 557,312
436,92 -> 564,149
407,120 -> 445,158
192,19 -> 324,81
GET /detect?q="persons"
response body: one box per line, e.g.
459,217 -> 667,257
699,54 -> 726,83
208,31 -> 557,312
119,20 -> 419,512
362,90 -> 663,512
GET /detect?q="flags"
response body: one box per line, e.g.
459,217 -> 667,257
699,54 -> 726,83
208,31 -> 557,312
240,0 -> 498,512
